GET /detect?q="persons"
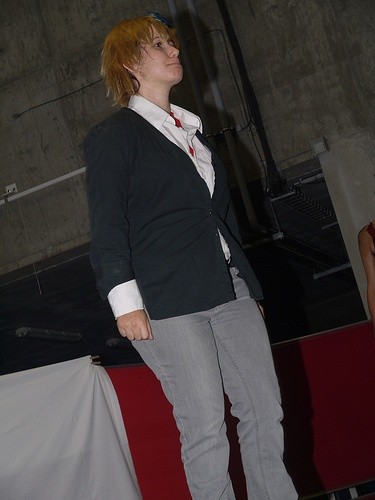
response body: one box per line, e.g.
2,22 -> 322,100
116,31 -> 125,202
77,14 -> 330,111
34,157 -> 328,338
80,11 -> 306,499
